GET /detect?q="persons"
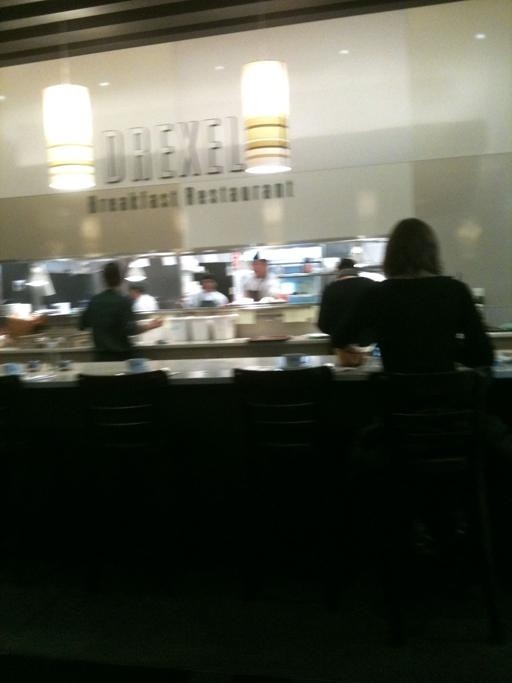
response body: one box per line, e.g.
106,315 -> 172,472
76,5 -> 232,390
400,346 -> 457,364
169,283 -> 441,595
175,271 -> 230,309
127,282 -> 162,314
332,218 -> 497,406
316,257 -> 383,356
74,260 -> 164,362
1,302 -> 48,349
240,251 -> 288,305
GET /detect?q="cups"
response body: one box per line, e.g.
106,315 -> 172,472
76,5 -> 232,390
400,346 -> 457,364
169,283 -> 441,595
56,359 -> 71,367
28,360 -> 39,367
128,358 -> 149,368
284,352 -> 307,365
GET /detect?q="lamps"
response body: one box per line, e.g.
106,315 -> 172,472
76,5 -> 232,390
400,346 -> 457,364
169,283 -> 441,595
43,82 -> 98,195
241,58 -> 293,177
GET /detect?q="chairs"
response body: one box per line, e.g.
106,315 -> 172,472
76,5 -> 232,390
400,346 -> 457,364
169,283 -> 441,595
230,364 -> 339,602
1,374 -> 64,558
74,369 -> 175,577
374,359 -> 501,648
